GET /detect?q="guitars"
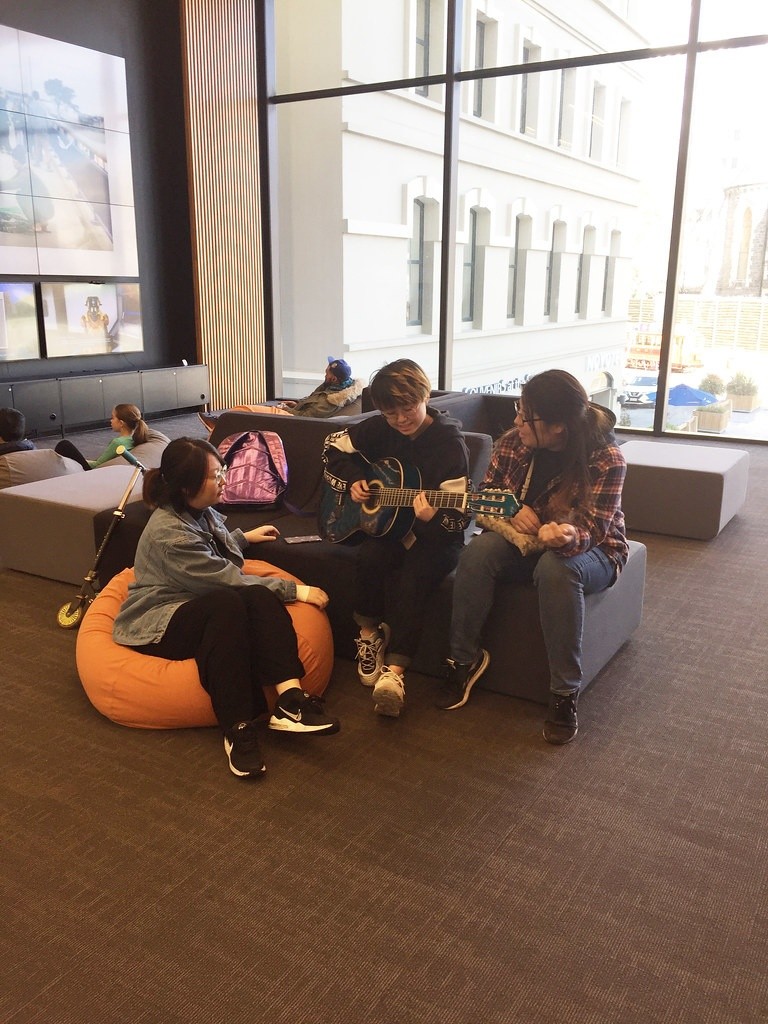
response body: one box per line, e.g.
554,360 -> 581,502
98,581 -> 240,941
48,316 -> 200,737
314,450 -> 524,545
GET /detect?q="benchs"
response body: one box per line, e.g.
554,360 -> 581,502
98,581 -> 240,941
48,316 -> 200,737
614,439 -> 750,541
0,465 -> 144,586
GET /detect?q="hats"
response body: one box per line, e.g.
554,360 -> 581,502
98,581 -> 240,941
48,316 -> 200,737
328,356 -> 351,381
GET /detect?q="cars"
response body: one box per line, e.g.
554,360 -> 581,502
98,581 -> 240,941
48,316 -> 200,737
620,373 -> 658,409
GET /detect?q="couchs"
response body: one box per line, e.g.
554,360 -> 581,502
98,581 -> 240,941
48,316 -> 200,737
94,386 -> 647,709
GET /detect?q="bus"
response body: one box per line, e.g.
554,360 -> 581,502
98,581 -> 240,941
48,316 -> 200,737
625,331 -> 686,373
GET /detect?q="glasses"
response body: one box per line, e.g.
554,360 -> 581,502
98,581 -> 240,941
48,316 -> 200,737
380,401 -> 423,421
514,398 -> 543,422
207,465 -> 227,480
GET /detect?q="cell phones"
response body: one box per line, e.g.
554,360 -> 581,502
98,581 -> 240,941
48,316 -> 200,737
284,535 -> 322,544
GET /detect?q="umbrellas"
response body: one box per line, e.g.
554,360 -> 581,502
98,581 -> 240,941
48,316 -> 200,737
644,384 -> 717,406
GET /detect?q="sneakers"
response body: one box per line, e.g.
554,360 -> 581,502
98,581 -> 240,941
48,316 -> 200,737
268,695 -> 340,736
372,666 -> 404,717
543,687 -> 580,745
437,649 -> 490,709
224,720 -> 266,778
353,623 -> 390,685
198,412 -> 218,433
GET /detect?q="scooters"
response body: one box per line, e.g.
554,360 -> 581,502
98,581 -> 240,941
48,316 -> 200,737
57,445 -> 151,629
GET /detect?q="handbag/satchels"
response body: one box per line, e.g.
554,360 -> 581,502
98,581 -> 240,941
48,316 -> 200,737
476,512 -> 545,557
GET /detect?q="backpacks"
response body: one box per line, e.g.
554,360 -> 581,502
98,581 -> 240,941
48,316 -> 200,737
216,430 -> 288,504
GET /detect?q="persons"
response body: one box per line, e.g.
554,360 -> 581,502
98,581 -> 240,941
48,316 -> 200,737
318,360 -> 470,716
87,402 -> 149,470
113,436 -> 341,782
198,357 -> 354,433
0,407 -> 38,457
0,78 -> 90,233
433,370 -> 630,746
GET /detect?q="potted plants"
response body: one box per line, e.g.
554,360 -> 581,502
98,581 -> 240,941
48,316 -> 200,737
691,374 -> 732,434
725,373 -> 761,412
